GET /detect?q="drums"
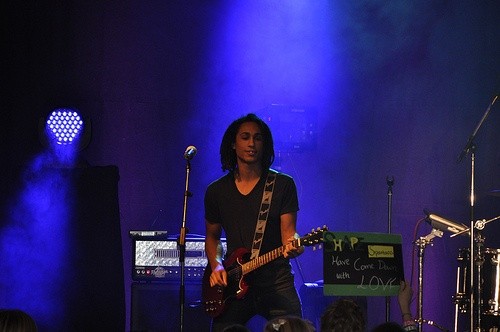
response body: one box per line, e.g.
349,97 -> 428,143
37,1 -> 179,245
454,248 -> 471,316
474,247 -> 500,318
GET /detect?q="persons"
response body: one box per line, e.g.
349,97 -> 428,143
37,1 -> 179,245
204,114 -> 304,332
0,309 -> 36,332
222,279 -> 419,332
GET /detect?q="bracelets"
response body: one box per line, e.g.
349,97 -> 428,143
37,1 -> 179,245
401,313 -> 417,331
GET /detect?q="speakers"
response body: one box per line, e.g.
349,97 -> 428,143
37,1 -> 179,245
130,281 -> 212,332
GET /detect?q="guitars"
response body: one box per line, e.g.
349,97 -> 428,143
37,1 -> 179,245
201,223 -> 330,320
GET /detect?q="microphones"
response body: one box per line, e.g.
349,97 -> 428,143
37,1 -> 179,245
184,146 -> 198,160
